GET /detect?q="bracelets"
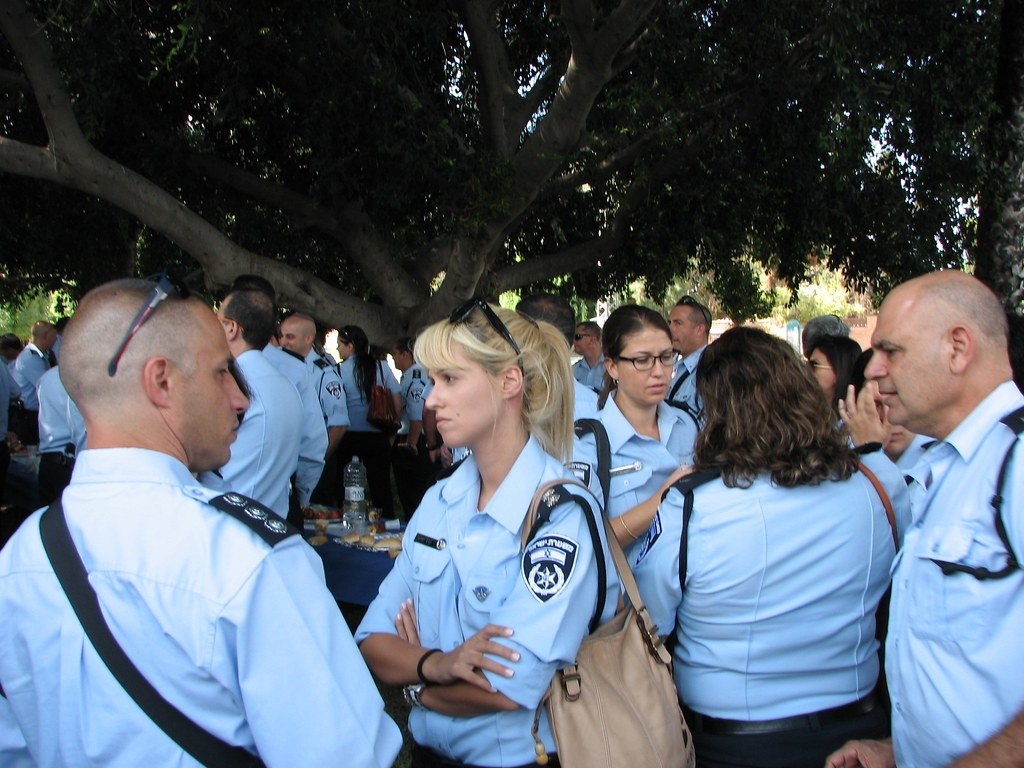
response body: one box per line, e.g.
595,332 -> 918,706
620,515 -> 638,539
417,648 -> 443,681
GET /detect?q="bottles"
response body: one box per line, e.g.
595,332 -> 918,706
343,456 -> 365,533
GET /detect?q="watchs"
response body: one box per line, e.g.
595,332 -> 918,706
404,684 -> 429,710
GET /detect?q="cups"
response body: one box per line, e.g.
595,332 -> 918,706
385,519 -> 400,531
27,445 -> 37,460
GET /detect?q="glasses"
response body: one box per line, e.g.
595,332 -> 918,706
807,360 -> 834,374
677,295 -> 711,329
337,327 -> 357,348
449,297 -> 525,377
108,270 -> 191,376
574,333 -> 592,343
43,322 -> 52,336
612,349 -> 682,372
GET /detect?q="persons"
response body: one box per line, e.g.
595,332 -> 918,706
515,292 -> 927,637
0,274 -> 473,532
0,278 -> 403,768
353,297 -> 619,768
825,269 -> 1024,768
622,327 -> 895,768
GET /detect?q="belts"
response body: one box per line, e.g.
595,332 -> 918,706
681,691 -> 884,735
411,746 -> 464,768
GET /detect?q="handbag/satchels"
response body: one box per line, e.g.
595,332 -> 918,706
521,479 -> 697,768
8,399 -> 41,445
366,361 -> 402,432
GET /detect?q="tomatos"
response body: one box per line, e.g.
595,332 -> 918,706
304,509 -> 343,520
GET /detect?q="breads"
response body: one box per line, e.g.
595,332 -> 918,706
309,519 -> 403,551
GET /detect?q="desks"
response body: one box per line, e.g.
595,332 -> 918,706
300,516 -> 409,628
